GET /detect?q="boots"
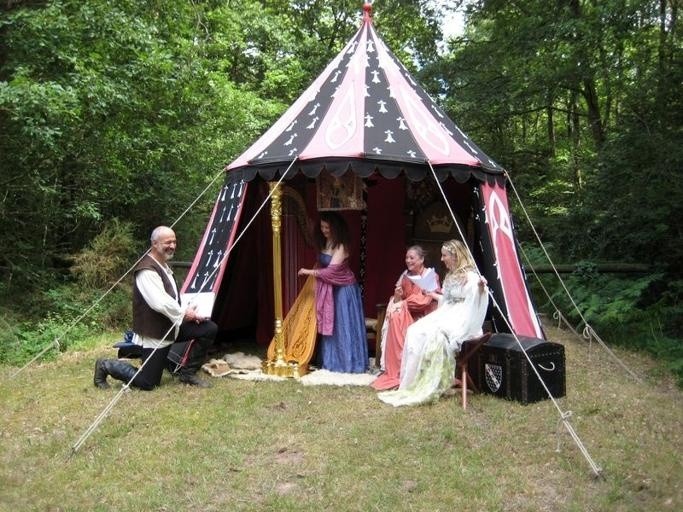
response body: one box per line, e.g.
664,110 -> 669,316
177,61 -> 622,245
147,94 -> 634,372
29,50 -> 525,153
93,358 -> 136,388
181,337 -> 214,395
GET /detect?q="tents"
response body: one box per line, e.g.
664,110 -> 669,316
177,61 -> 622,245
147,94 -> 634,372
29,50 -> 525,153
167,3 -> 547,375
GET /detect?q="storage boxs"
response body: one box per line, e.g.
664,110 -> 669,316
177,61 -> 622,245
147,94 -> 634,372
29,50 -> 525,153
470,333 -> 566,404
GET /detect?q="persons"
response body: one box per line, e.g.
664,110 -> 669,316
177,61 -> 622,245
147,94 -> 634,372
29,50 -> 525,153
94,225 -> 217,389
371,244 -> 440,392
377,239 -> 490,408
297,211 -> 370,373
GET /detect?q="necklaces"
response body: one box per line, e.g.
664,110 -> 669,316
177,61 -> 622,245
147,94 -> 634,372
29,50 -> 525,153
324,240 -> 334,251
